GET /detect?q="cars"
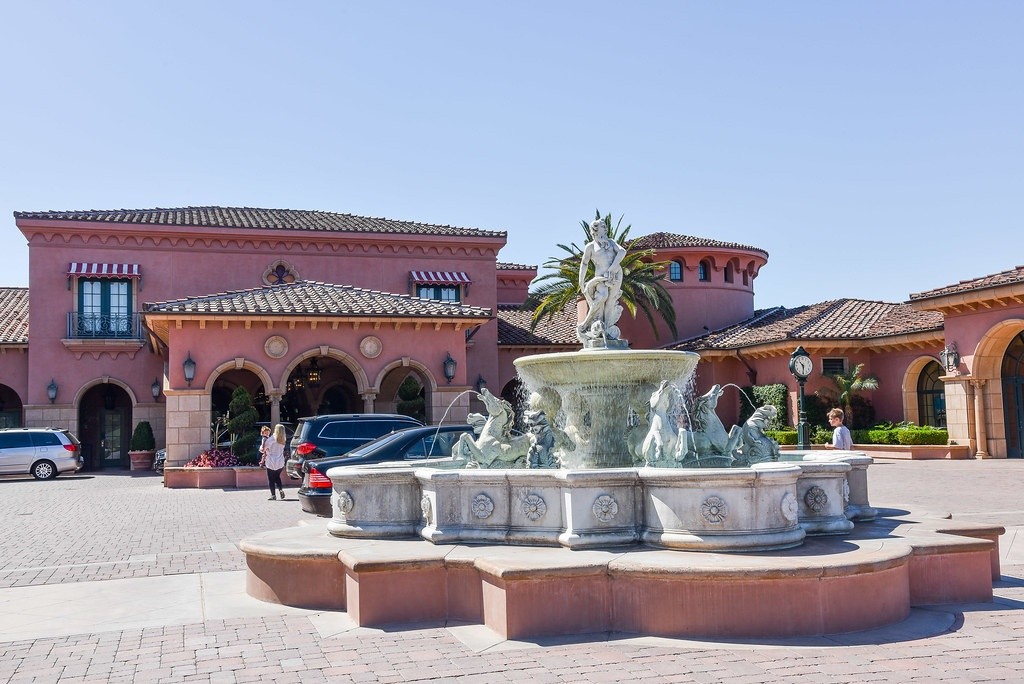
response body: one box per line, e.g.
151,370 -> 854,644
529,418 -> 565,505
0,426 -> 86,480
298,423 -> 524,520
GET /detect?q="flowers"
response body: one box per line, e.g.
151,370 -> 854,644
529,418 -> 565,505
182,449 -> 241,467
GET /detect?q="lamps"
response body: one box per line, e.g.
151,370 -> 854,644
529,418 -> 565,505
939,341 -> 961,377
476,374 -> 486,394
151,376 -> 162,402
46,378 -> 58,404
443,353 -> 459,384
182,349 -> 195,388
263,357 -> 321,406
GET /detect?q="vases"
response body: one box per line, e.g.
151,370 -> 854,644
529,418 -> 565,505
164,467 -> 236,489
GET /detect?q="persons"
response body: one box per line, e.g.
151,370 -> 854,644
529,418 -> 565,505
259,424 -> 286,500
578,218 -> 627,331
825,408 -> 853,450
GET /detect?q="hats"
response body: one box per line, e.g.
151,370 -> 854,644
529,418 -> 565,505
260,425 -> 271,437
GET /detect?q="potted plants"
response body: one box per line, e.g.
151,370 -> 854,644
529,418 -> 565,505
224,385 -> 302,487
128,421 -> 156,470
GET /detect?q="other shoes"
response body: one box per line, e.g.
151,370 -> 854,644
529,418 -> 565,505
260,464 -> 264,469
280,491 -> 285,499
268,496 -> 276,500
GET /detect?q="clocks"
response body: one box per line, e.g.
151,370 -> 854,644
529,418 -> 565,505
794,356 -> 812,375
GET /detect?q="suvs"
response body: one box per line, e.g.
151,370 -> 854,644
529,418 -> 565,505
285,412 -> 454,480
152,420 -> 297,475
